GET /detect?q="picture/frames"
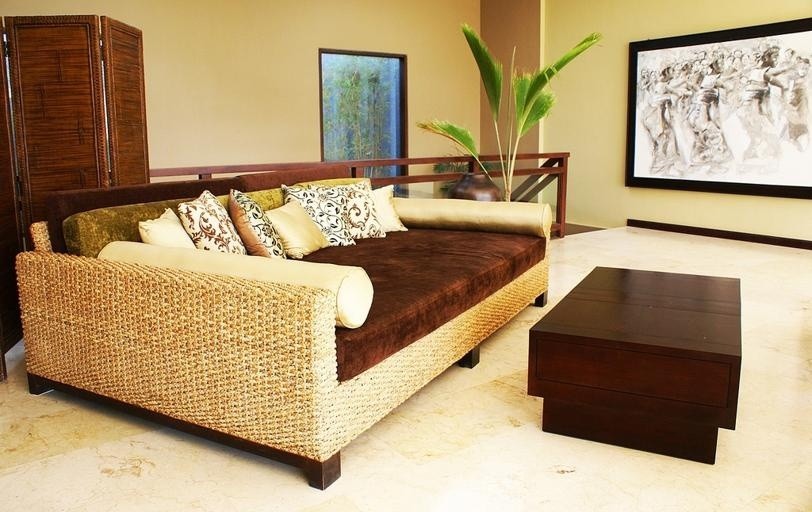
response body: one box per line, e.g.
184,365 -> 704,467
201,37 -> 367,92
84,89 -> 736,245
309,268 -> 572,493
624,18 -> 811,201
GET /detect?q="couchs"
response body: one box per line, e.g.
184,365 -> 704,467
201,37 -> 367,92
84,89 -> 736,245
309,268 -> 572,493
16,165 -> 553,490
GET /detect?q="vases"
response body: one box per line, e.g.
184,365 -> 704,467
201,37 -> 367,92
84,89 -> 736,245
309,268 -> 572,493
448,173 -> 502,202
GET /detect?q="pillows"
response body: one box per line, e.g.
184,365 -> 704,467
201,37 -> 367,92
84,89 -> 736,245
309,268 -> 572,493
178,189 -> 249,255
373,183 -> 409,233
280,182 -> 358,248
308,179 -> 387,240
228,188 -> 289,260
265,200 -> 333,259
137,208 -> 198,250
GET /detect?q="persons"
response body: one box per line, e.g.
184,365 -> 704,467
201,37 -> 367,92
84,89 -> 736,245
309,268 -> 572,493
634,30 -> 812,186
